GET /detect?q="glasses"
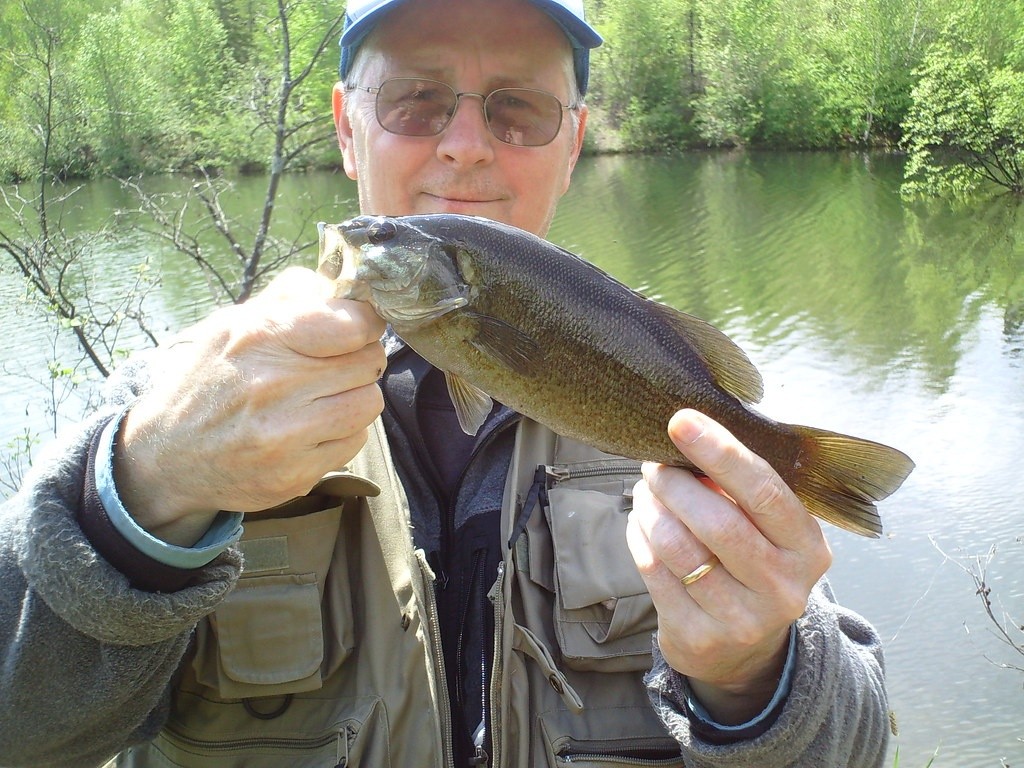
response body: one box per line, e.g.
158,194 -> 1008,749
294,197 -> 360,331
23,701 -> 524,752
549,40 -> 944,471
348,78 -> 577,147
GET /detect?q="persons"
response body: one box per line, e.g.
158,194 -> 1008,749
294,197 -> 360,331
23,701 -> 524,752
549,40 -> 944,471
0,0 -> 897,767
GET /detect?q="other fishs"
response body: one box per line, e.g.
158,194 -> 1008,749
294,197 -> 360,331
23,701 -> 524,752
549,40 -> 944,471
317,213 -> 915,539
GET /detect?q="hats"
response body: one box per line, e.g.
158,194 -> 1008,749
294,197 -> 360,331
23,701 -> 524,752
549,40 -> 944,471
338,1 -> 603,104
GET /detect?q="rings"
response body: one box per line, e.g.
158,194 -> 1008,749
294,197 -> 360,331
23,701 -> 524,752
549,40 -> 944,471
681,556 -> 720,587
